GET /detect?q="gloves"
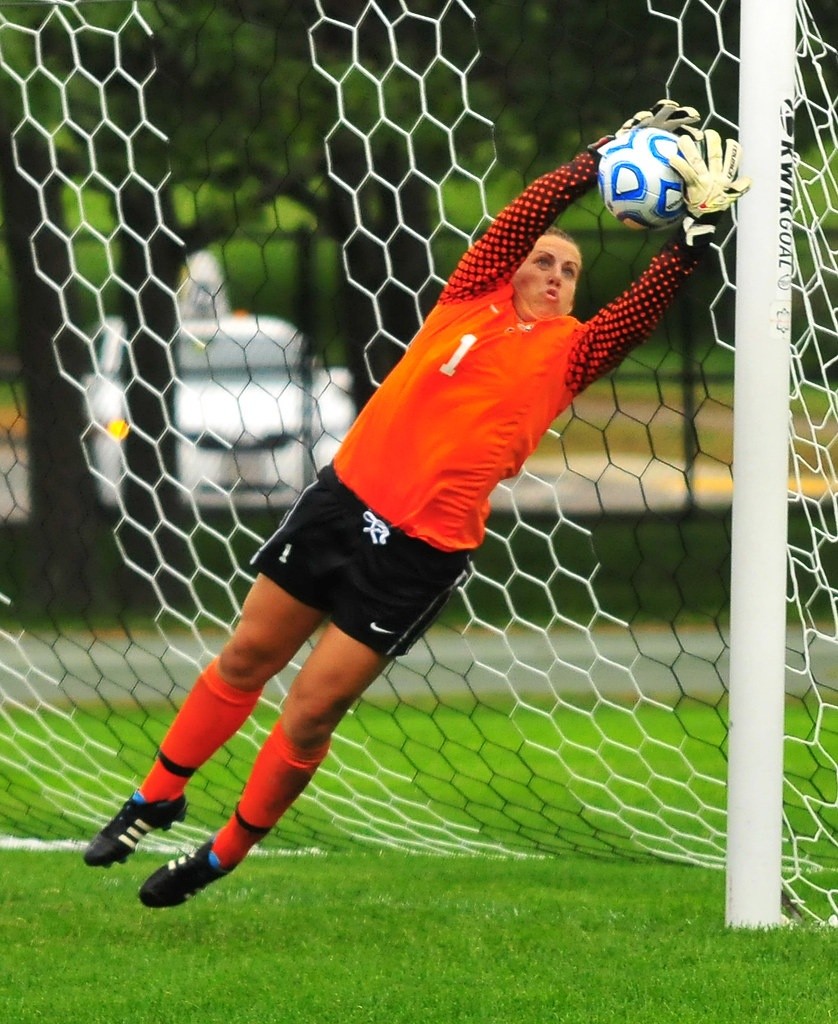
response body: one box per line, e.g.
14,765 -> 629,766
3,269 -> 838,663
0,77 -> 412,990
588,100 -> 705,161
666,128 -> 751,253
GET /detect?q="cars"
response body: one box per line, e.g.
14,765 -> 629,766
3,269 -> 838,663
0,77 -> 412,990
81,247 -> 359,511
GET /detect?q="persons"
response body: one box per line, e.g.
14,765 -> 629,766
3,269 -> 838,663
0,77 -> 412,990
82,98 -> 753,907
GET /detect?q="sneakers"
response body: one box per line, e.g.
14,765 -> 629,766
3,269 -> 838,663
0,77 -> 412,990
140,832 -> 234,908
83,788 -> 189,869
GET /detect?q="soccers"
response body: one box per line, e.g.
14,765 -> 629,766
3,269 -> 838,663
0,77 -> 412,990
597,126 -> 687,231
